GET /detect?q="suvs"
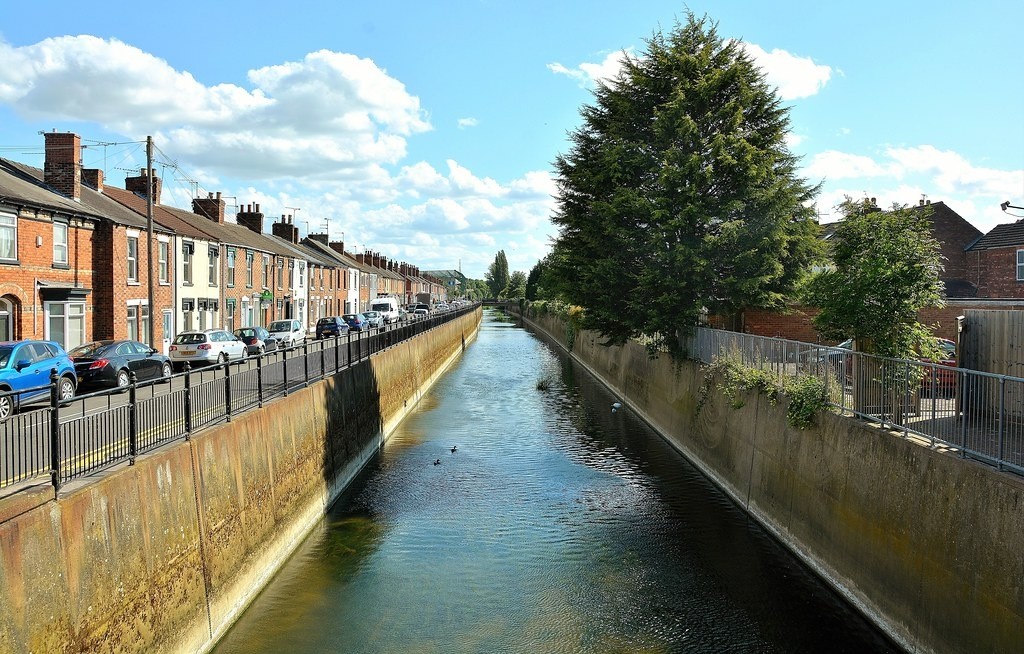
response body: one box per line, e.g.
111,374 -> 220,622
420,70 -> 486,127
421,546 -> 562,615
169,328 -> 249,373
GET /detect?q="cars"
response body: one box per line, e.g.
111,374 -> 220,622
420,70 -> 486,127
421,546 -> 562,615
0,341 -> 78,424
435,300 -> 470,311
342,314 -> 371,333
233,327 -> 278,357
316,316 -> 351,340
267,319 -> 308,350
406,301 -> 423,313
66,340 -> 175,394
795,332 -> 956,392
411,309 -> 429,320
362,311 -> 385,328
398,308 -> 408,322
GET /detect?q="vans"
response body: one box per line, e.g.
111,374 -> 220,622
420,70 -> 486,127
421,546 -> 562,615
414,304 -> 432,315
371,297 -> 400,323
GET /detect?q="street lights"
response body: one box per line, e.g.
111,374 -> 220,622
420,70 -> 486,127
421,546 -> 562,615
400,265 -> 405,308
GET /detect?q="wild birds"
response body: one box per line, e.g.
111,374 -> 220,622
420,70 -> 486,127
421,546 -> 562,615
434,459 -> 440,465
451,446 -> 457,452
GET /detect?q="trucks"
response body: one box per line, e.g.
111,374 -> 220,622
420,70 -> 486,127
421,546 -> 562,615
416,292 -> 435,307
